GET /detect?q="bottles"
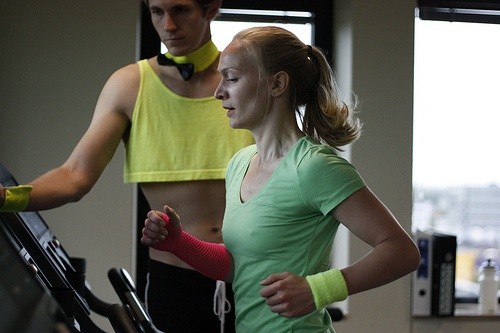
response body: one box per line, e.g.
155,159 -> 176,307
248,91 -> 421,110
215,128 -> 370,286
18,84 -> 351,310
478,258 -> 500,313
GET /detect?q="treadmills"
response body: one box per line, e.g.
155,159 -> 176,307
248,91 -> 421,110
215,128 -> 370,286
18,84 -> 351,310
0,162 -> 163,333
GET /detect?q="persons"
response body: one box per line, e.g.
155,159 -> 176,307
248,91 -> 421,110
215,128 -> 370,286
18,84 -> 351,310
0,0 -> 257,333
140,27 -> 420,333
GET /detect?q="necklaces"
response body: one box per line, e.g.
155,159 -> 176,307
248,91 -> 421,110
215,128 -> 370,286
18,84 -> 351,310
156,39 -> 218,80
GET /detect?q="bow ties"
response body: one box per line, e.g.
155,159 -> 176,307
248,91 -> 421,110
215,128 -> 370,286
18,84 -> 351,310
157,51 -> 195,81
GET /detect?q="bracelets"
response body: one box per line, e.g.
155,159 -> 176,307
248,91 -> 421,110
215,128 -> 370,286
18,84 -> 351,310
305,268 -> 347,311
3,185 -> 33,212
